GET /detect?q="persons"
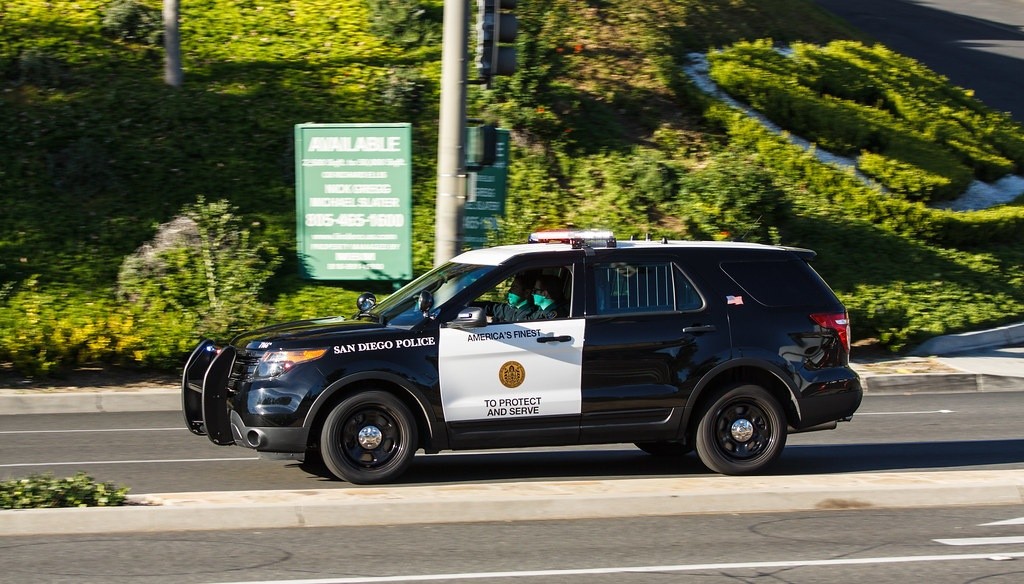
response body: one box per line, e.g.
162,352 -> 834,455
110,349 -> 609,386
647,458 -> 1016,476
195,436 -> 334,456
526,275 -> 568,320
464,274 -> 538,323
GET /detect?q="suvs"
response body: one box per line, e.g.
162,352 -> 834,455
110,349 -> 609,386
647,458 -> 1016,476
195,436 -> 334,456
180,231 -> 864,487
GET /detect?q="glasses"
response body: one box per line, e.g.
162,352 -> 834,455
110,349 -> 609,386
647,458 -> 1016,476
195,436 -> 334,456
532,287 -> 544,294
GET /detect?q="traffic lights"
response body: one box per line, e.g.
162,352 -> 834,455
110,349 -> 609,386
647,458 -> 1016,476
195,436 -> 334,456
476,0 -> 522,81
